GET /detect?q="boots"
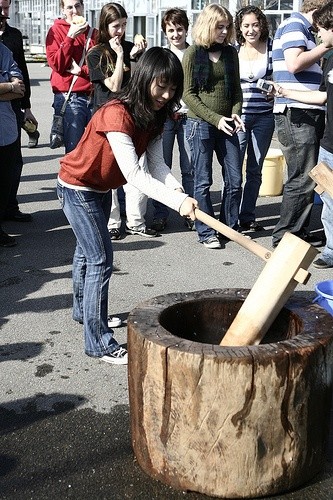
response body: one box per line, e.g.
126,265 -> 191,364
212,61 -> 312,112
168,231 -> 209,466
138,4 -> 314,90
22,118 -> 40,148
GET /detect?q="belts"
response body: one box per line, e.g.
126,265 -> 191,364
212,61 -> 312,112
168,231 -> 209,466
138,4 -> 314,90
171,112 -> 187,119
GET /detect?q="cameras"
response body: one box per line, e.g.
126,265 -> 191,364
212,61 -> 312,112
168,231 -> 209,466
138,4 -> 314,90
256,78 -> 273,93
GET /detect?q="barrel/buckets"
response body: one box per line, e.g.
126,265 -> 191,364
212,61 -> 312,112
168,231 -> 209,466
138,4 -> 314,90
314,193 -> 322,204
244,148 -> 285,196
312,279 -> 333,317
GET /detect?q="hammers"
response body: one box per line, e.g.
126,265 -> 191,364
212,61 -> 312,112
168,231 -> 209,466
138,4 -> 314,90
193,207 -> 319,347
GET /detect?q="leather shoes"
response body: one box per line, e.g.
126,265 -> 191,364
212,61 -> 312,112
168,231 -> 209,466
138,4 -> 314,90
4,209 -> 32,222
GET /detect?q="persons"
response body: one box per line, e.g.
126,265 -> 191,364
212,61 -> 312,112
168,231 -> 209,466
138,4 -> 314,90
58,46 -> 199,364
46,0 -> 333,268
0,0 -> 38,247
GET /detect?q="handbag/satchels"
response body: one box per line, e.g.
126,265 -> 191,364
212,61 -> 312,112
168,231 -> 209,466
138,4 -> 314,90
49,114 -> 64,149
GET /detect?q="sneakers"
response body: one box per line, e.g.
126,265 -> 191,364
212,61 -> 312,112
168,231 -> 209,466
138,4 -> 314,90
107,315 -> 122,327
0,229 -> 17,247
122,219 -> 157,238
184,216 -> 197,231
91,346 -> 128,365
150,205 -> 169,232
241,219 -> 265,234
313,258 -> 331,268
198,233 -> 221,249
292,227 -> 323,246
107,217 -> 124,240
